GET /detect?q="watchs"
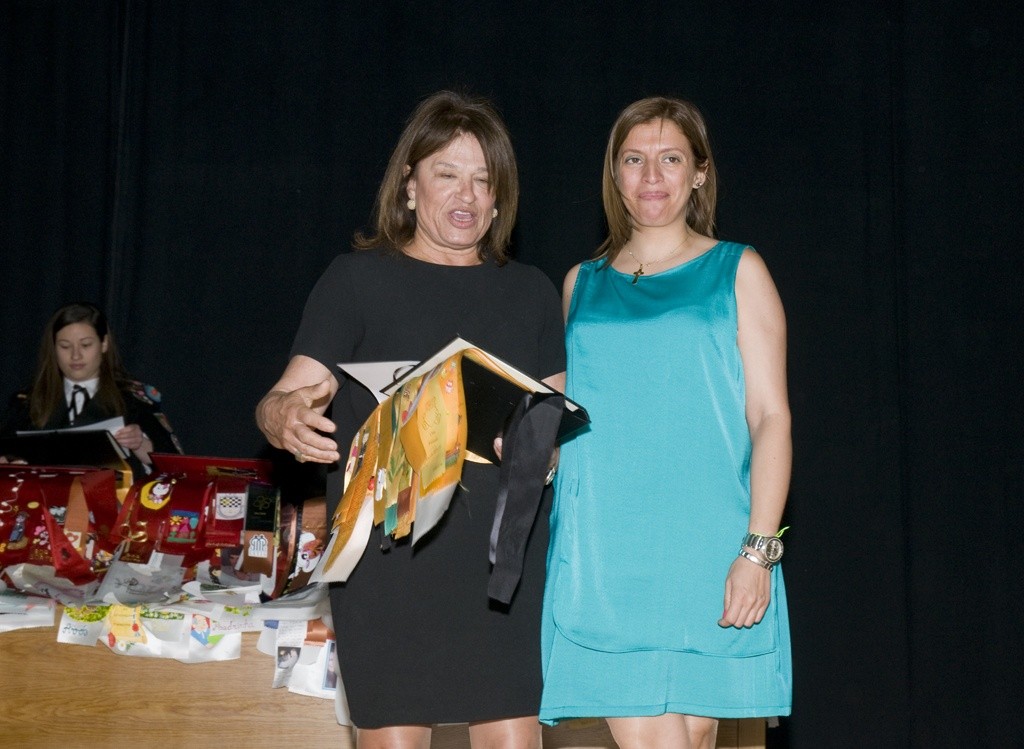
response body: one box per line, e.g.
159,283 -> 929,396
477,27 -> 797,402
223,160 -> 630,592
741,532 -> 784,563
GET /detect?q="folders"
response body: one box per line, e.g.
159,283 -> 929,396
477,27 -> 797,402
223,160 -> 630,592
379,336 -> 591,467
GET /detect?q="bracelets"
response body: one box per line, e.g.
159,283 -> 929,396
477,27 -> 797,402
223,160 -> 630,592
738,548 -> 773,572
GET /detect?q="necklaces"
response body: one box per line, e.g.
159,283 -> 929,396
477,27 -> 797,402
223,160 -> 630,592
625,235 -> 694,285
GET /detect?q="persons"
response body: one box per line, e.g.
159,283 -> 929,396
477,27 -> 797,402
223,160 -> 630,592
0,303 -> 184,484
539,98 -> 792,749
255,94 -> 567,749
